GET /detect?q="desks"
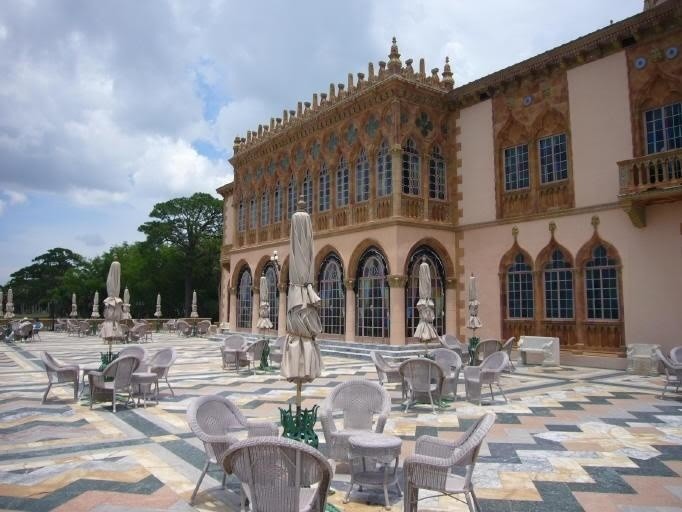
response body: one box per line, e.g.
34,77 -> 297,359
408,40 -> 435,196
342,432 -> 404,510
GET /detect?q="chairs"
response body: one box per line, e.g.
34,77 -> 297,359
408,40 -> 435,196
402,411 -> 496,509
187,393 -> 280,505
655,346 -> 682,400
2,317 -> 231,344
41,345 -> 174,412
222,436 -> 332,512
321,379 -> 391,472
368,334 -> 518,416
218,333 -> 293,373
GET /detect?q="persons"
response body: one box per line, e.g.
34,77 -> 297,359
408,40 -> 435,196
5,317 -> 32,343
24,318 -> 40,340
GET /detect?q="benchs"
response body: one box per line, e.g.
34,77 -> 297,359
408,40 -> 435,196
627,343 -> 661,377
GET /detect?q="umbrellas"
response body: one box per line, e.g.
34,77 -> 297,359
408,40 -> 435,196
257,269 -> 274,336
153,292 -> 163,319
466,276 -> 483,336
190,289 -> 199,318
69,292 -> 78,317
413,254 -> 438,343
280,193 -> 323,413
90,290 -> 100,317
121,282 -> 132,324
4,286 -> 15,319
99,253 -> 125,363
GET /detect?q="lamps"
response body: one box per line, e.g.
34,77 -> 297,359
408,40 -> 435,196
271,251 -> 280,271
